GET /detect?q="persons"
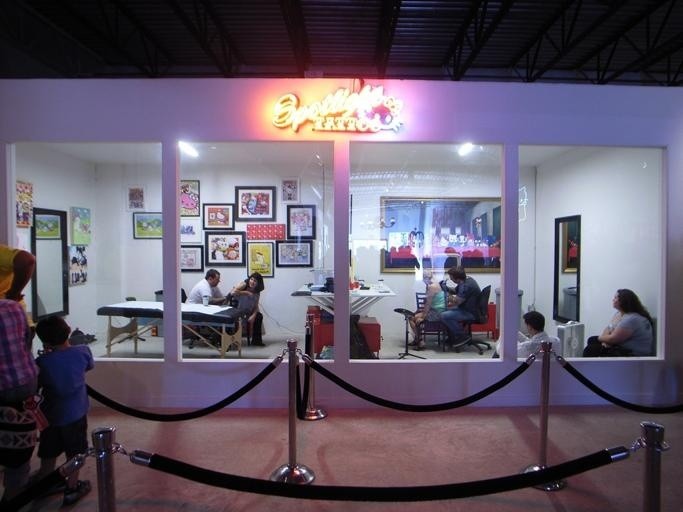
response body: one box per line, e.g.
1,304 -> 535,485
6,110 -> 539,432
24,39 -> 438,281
492,313 -> 562,360
183,269 -> 226,308
582,289 -> 655,357
407,269 -> 446,351
35,310 -> 95,511
0,296 -> 40,511
231,273 -> 265,349
0,242 -> 37,350
439,266 -> 481,349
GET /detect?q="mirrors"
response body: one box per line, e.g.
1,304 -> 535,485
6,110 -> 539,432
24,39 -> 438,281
29,206 -> 68,318
552,213 -> 581,326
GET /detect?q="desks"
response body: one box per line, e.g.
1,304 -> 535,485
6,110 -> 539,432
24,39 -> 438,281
97,301 -> 242,361
291,279 -> 396,359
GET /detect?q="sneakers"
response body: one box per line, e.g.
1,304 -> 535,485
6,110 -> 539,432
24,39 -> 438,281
452,335 -> 471,348
63,479 -> 91,505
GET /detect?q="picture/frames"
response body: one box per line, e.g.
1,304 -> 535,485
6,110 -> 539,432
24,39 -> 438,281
378,193 -> 500,274
127,184 -> 146,212
561,221 -> 578,273
35,215 -> 60,240
178,174 -> 318,279
131,211 -> 163,239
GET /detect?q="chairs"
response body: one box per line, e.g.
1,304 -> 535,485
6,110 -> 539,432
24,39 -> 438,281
416,293 -> 446,345
227,293 -> 251,346
153,286 -> 207,348
453,286 -> 494,357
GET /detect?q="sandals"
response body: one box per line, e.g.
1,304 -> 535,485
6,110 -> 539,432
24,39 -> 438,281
407,336 -> 426,350
